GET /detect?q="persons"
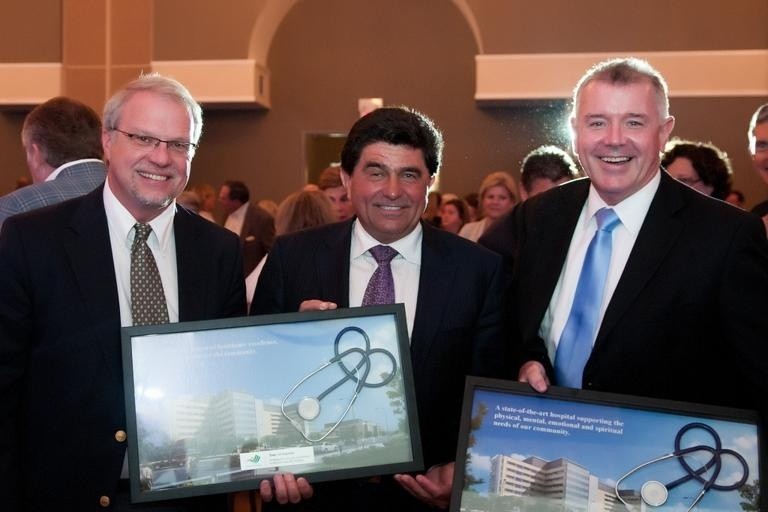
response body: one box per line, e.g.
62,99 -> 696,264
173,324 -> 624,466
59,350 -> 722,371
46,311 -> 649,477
250,104 -> 513,511
176,104 -> 768,304
476,58 -> 767,511
0,67 -> 248,510
0,95 -> 107,223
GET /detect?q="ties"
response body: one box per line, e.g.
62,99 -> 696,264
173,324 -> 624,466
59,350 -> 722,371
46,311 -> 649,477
131,224 -> 168,325
552,208 -> 623,391
362,246 -> 399,305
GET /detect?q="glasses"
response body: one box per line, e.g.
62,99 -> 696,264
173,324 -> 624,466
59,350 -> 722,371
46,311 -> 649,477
113,128 -> 198,155
677,178 -> 701,186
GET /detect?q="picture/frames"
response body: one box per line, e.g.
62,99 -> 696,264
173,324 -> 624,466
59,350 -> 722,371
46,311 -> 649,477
450,377 -> 768,512
121,303 -> 425,503
